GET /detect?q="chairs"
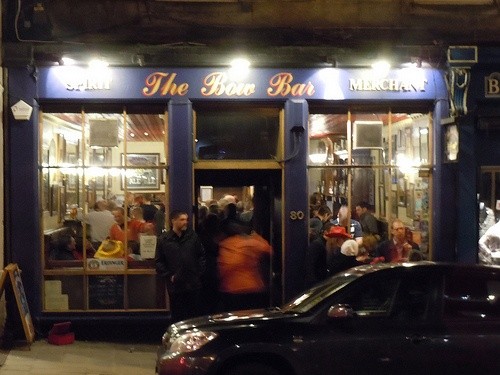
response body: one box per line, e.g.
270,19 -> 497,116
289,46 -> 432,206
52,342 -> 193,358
124,260 -> 158,309
51,260 -> 91,309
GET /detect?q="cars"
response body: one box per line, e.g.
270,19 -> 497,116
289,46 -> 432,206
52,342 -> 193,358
155,260 -> 500,375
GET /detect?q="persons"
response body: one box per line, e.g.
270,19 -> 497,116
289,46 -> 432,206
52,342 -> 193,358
199,203 -> 275,311
46,196 -> 158,261
307,190 -> 382,289
374,217 -> 415,265
151,210 -> 206,322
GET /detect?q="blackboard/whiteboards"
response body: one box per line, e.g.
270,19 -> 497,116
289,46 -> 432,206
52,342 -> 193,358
4,262 -> 36,341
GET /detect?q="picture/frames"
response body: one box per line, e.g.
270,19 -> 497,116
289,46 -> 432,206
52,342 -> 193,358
37,133 -> 114,225
120,152 -> 160,190
367,123 -> 430,222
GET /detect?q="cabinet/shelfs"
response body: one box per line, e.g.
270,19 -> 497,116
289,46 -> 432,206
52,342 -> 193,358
316,133 -> 370,236
